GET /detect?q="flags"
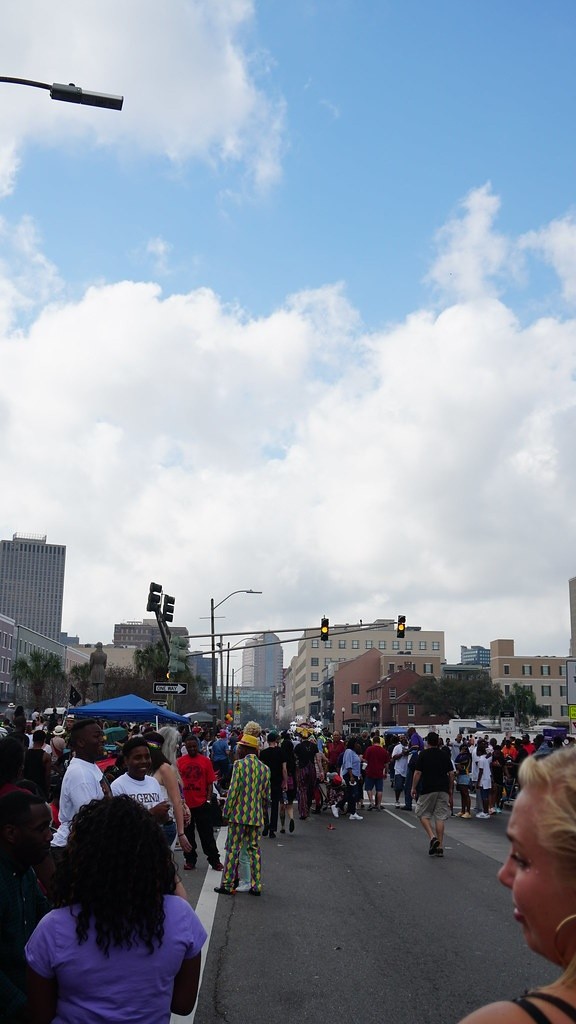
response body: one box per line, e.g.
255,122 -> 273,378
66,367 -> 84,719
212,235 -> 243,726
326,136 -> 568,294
69,685 -> 82,707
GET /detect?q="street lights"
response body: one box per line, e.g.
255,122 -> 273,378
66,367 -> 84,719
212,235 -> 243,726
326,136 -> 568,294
210,589 -> 262,721
372,705 -> 378,723
238,680 -> 253,704
231,665 -> 259,717
341,706 -> 345,726
332,709 -> 336,732
226,638 -> 264,722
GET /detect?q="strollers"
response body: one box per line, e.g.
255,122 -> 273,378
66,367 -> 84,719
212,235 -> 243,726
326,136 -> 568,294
499,756 -> 521,811
315,775 -> 350,815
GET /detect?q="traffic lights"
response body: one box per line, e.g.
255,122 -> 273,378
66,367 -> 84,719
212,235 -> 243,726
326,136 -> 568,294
321,618 -> 329,641
397,615 -> 406,638
163,594 -> 175,622
147,582 -> 162,611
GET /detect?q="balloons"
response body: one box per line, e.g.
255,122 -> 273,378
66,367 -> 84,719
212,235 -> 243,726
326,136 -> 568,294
224,710 -> 233,724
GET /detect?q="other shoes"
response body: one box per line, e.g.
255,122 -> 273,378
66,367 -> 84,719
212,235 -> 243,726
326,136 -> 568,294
367,805 -> 373,811
434,847 -> 444,857
184,862 -> 194,869
394,801 -> 399,808
374,804 -> 384,810
312,809 -> 321,814
428,837 -> 439,855
214,885 -> 234,896
213,864 -> 223,871
249,887 -> 261,896
402,806 -> 413,810
262,819 -> 295,838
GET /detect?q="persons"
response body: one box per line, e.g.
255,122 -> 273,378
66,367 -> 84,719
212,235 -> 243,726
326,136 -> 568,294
89,641 -> 107,702
458,738 -> 576,1024
411,732 -> 454,857
23,792 -> 207,1024
388,727 -> 576,818
0,720 -> 270,903
0,790 -> 53,1024
3,702 -> 59,725
260,727 -> 390,838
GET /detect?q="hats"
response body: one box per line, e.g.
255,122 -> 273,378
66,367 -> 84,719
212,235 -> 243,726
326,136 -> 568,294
8,703 -> 15,707
236,733 -> 261,748
53,725 -> 65,735
219,731 -> 227,738
64,714 -> 75,720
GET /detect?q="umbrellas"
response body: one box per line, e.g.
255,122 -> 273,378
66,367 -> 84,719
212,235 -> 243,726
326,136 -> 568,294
384,727 -> 408,736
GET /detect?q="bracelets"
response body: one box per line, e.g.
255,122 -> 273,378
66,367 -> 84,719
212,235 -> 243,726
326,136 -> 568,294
178,833 -> 185,837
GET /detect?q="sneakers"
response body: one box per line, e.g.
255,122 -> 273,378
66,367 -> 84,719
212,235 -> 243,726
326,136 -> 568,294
454,806 -> 502,819
349,813 -> 363,820
331,803 -> 339,818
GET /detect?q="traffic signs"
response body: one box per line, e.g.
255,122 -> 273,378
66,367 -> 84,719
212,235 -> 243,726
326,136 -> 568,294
152,681 -> 187,695
151,698 -> 169,706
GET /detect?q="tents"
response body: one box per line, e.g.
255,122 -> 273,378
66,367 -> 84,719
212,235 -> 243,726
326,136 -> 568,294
62,694 -> 191,732
